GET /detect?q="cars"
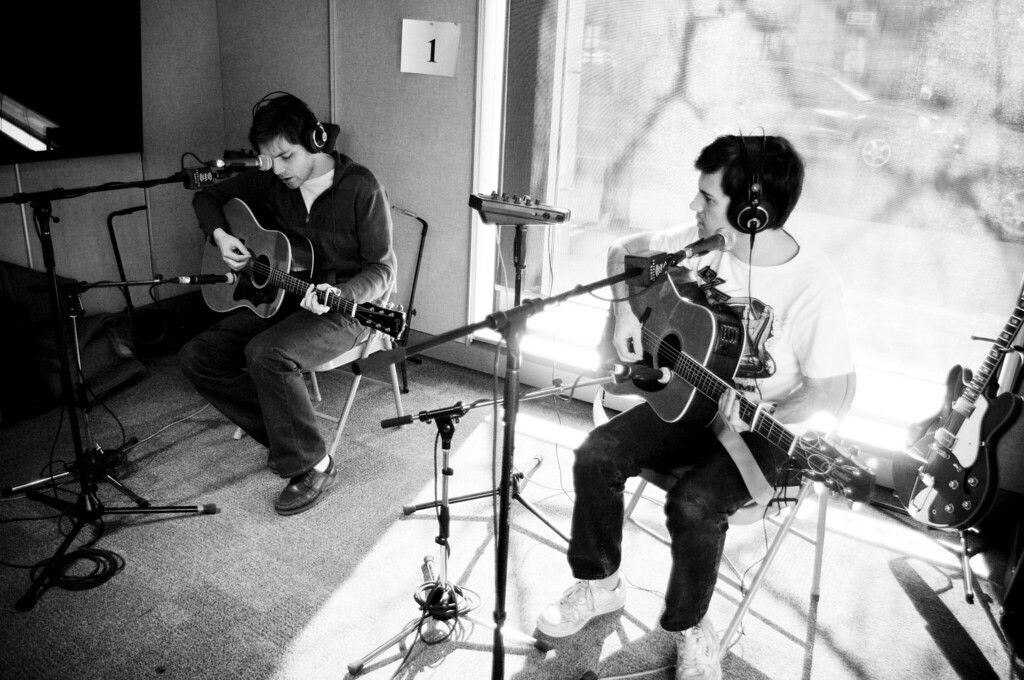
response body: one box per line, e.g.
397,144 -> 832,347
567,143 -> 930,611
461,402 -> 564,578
730,60 -> 1024,242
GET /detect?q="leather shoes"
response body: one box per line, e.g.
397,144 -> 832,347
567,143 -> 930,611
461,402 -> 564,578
267,448 -> 280,474
275,454 -> 337,516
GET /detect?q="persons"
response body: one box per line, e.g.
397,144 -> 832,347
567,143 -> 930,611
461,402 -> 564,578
176,93 -> 400,515
536,134 -> 857,680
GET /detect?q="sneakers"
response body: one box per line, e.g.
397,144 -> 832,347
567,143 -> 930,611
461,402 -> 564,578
671,613 -> 721,680
536,572 -> 625,637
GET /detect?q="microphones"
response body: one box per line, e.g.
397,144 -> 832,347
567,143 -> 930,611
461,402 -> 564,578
675,228 -> 736,261
210,155 -> 272,171
172,272 -> 237,284
610,364 -> 674,383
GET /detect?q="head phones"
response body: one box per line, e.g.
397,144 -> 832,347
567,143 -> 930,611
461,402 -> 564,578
727,125 -> 775,233
252,90 -> 328,154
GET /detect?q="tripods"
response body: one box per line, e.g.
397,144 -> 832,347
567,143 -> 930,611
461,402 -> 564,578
345,225 -> 573,674
0,163 -> 226,612
867,336 -> 1014,600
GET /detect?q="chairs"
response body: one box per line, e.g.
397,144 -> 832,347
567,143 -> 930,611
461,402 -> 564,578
232,253 -> 403,458
621,368 -> 856,680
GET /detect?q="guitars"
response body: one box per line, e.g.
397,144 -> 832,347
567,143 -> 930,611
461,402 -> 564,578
598,251 -> 876,505
200,196 -> 406,340
890,286 -> 1024,531
